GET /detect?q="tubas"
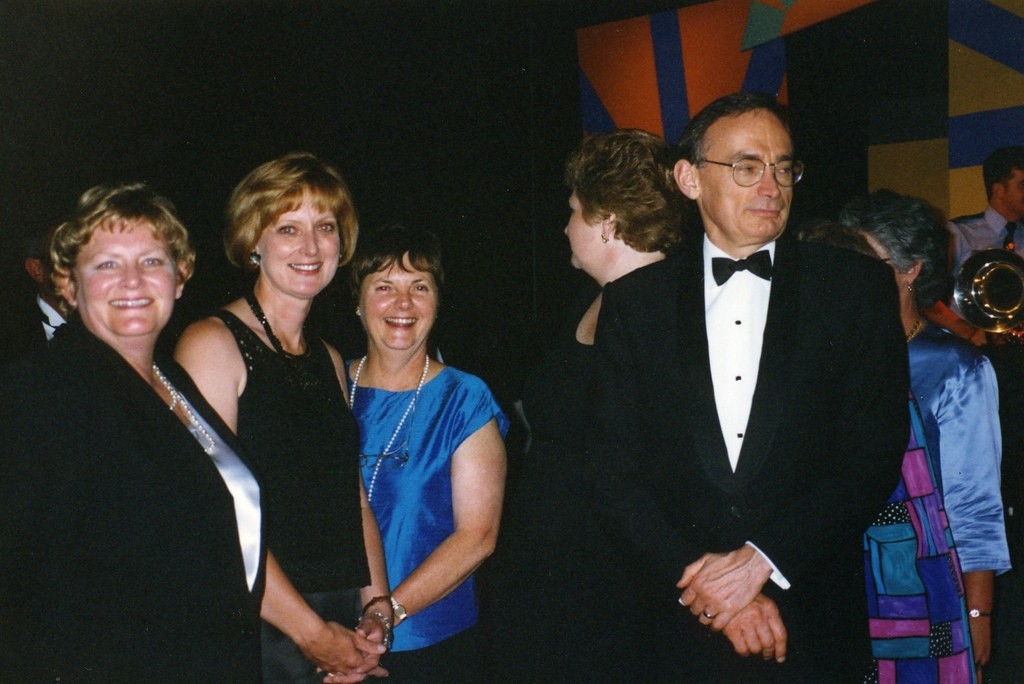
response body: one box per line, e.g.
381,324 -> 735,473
951,247 -> 1024,352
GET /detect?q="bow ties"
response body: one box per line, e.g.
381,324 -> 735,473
712,250 -> 772,286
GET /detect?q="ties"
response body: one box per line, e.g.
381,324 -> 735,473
1004,222 -> 1016,253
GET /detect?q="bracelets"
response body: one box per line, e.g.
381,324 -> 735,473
969,608 -> 991,618
356,611 -> 390,648
363,596 -> 390,612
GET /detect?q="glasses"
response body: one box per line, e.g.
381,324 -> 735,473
696,159 -> 804,187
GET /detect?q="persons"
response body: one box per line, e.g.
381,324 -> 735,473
521,130 -> 684,684
920,146 -> 1024,346
174,151 -> 394,684
839,190 -> 1013,684
344,227 -> 511,684
585,93 -> 909,684
792,218 -> 881,263
0,180 -> 271,684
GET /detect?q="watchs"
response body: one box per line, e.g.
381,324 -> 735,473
391,596 -> 424,619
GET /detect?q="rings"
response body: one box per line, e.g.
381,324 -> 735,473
704,611 -> 713,620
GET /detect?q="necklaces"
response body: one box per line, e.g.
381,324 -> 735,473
907,319 -> 925,342
153,362 -> 215,452
246,288 -> 312,360
349,355 -> 429,502
